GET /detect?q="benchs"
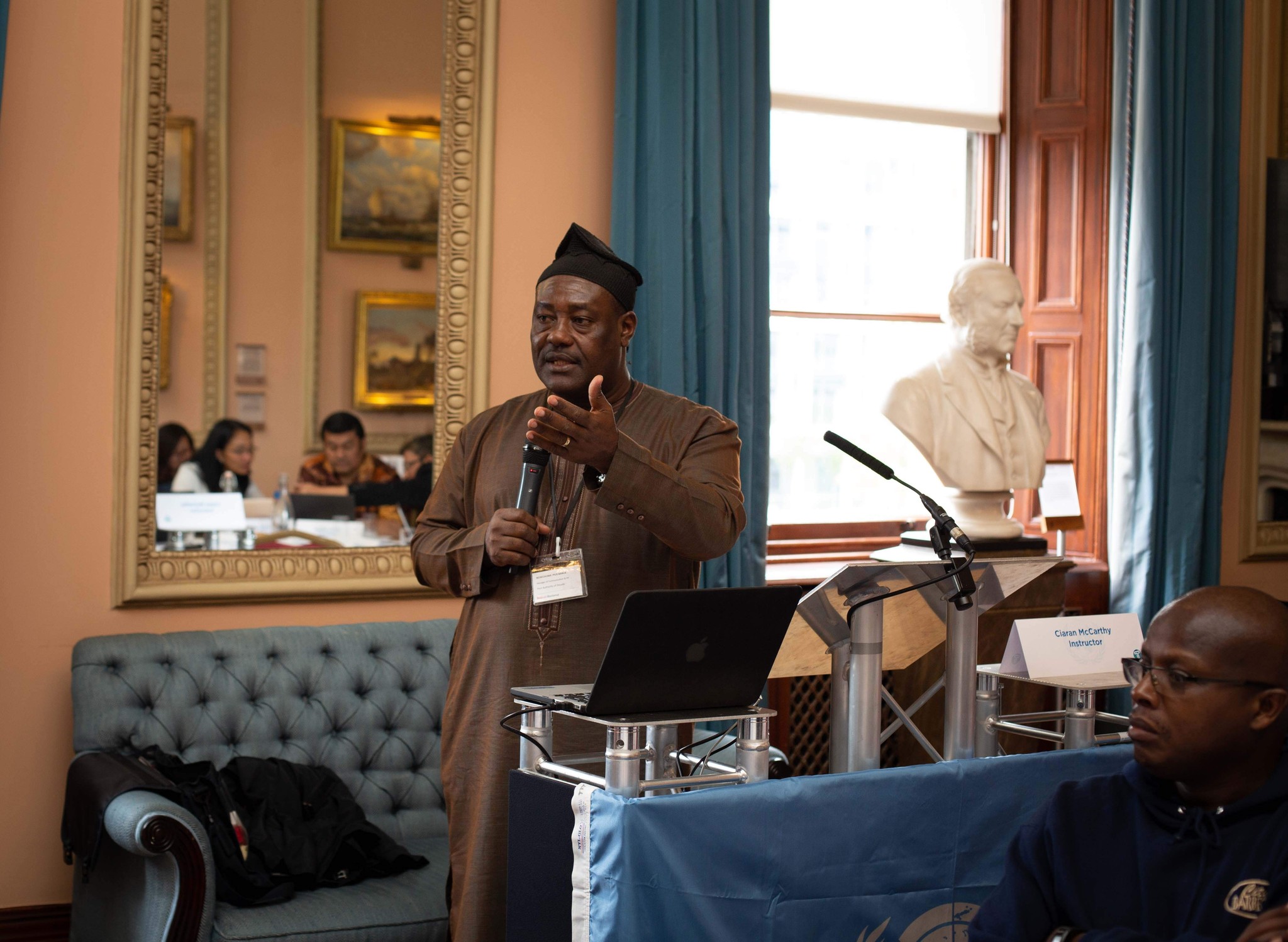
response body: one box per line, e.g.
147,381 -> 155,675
568,742 -> 1141,942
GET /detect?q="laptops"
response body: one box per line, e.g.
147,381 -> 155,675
290,493 -> 354,520
509,585 -> 802,716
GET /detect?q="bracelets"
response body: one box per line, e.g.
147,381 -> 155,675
596,473 -> 606,482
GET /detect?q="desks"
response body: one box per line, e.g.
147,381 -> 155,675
975,662 -> 1135,758
246,517 -> 415,551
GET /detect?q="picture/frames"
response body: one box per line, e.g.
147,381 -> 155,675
353,289 -> 441,406
323,114 -> 440,254
154,115 -> 195,240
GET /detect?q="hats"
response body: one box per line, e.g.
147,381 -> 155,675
536,222 -> 644,313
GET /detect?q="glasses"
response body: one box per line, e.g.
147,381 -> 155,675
403,457 -> 422,470
1119,658 -> 1284,700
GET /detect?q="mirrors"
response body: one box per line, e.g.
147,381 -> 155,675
114,0 -> 502,606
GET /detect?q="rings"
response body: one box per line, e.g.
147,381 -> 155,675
561,436 -> 570,447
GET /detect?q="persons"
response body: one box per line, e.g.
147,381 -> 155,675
966,584 -> 1288,942
409,221 -> 747,942
167,418 -> 264,543
156,422 -> 196,542
881,256 -> 1052,492
292,411 -> 433,540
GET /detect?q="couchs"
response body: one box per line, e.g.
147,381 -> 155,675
67,617 -> 460,942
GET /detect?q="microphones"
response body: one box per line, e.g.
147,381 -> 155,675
506,436 -> 552,575
823,430 -> 976,555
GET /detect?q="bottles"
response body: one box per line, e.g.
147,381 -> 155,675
272,475 -> 295,541
